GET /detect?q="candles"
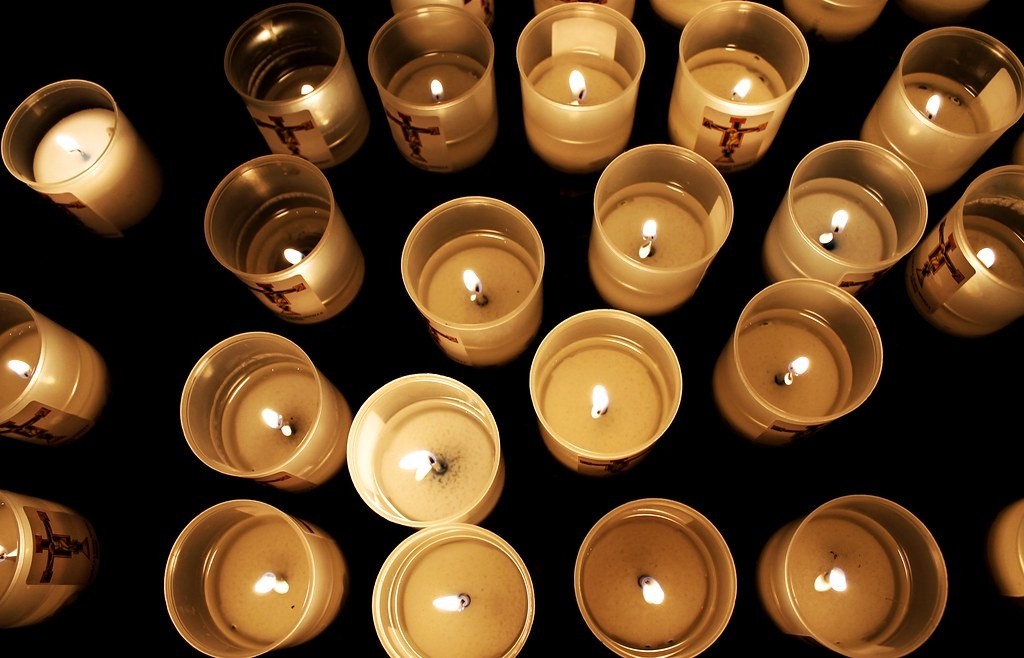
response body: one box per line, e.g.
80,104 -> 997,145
588,144 -> 735,318
573,498 -> 738,658
203,154 -> 366,324
761,140 -> 928,296
367,0 -> 499,173
347,375 -> 505,530
711,279 -> 884,446
650,0 -> 1024,197
223,2 -> 371,168
0,490 -> 99,629
372,523 -> 535,658
529,308 -> 683,478
0,293 -> 108,447
756,495 -> 1024,658
905,165 -> 1024,337
516,2 -> 646,174
179,332 -> 353,493
400,195 -> 546,366
164,499 -> 348,658
1,78 -> 165,239
